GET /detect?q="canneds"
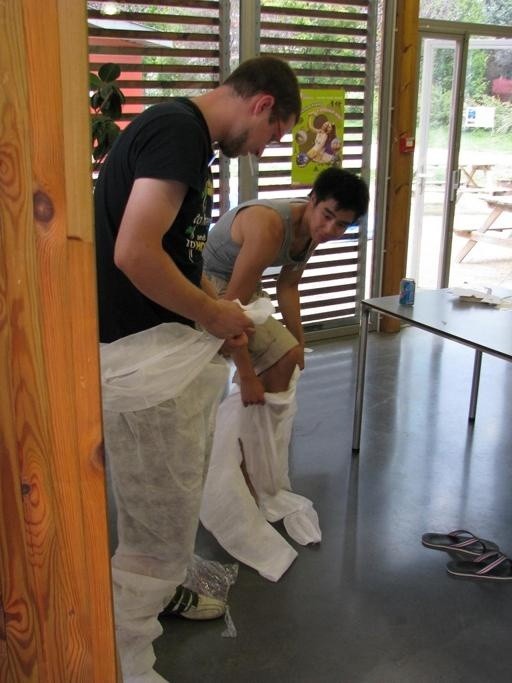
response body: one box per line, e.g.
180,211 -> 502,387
399,278 -> 415,305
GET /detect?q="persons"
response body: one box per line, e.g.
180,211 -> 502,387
200,166 -> 371,502
94,56 -> 302,682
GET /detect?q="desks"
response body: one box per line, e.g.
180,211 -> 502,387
455,195 -> 512,265
428,160 -> 495,200
350,287 -> 512,456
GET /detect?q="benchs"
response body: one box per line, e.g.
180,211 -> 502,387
453,224 -> 512,267
426,178 -> 511,204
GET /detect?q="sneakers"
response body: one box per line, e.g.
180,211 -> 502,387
166,585 -> 226,620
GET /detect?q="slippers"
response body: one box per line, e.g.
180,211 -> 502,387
421,529 -> 499,557
446,550 -> 512,580
